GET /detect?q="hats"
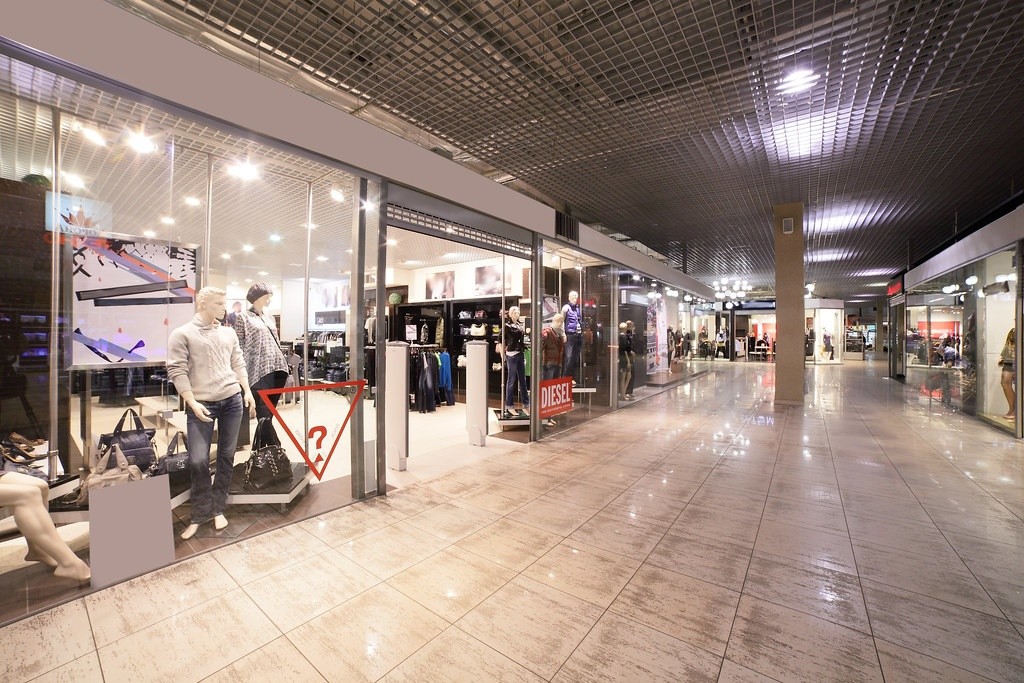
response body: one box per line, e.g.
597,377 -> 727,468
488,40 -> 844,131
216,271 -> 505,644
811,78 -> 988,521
247,282 -> 272,304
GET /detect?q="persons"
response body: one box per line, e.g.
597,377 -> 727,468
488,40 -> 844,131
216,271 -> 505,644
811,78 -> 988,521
668,325 -> 690,374
561,292 -> 584,384
167,287 -> 256,540
499,306 -> 530,414
0,470 -> 90,579
716,330 -> 727,358
619,320 -> 636,400
543,314 -> 564,426
228,302 -> 242,326
235,283 -> 289,445
998,328 -> 1016,419
823,329 -> 832,352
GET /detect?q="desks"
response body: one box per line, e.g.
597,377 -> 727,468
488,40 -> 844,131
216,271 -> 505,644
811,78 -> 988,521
134,394 -> 218,436
750,345 -> 776,361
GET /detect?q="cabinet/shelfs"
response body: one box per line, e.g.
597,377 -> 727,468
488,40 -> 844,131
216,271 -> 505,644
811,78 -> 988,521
394,300 -> 450,354
845,326 -> 864,361
450,296 -> 523,403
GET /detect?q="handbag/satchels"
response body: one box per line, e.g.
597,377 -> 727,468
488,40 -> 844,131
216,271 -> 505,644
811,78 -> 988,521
75,442 -> 147,507
0,431 -> 66,484
244,415 -> 293,492
98,409 -> 159,469
157,431 -> 192,489
